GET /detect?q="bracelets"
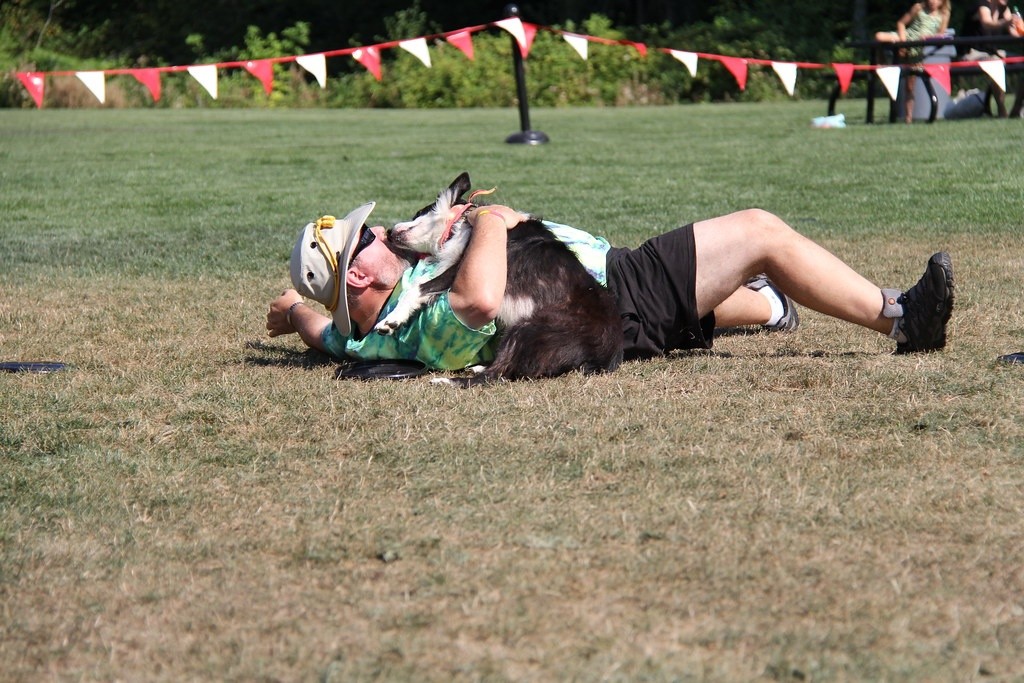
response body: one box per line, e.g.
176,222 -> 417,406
477,210 -> 506,222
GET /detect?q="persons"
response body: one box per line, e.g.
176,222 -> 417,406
876,0 -> 951,123
265,199 -> 957,370
960,0 -> 1024,118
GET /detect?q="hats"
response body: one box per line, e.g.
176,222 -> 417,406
289,201 -> 376,338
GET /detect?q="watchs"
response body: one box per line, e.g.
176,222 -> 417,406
286,302 -> 303,329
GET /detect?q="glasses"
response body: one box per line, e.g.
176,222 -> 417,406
348,222 -> 376,269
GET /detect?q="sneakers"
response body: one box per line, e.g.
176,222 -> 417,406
743,271 -> 799,334
895,251 -> 954,354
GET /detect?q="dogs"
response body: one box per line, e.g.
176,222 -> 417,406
383,173 -> 624,388
